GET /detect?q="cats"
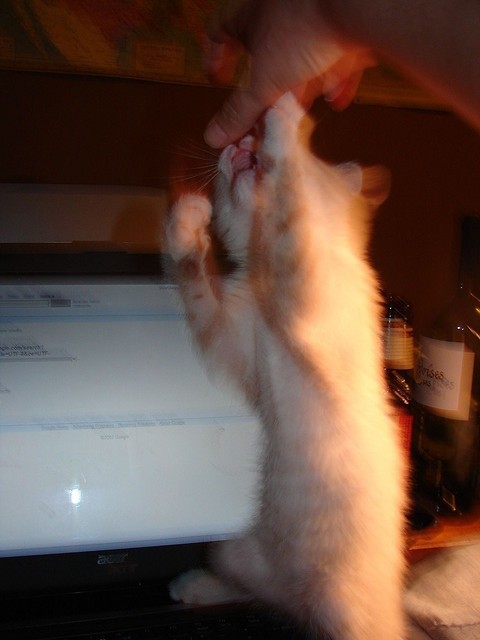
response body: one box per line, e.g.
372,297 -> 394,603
162,88 -> 416,635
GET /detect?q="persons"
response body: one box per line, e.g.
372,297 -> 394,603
205,0 -> 480,149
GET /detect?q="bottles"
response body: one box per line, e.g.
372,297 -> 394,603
414,213 -> 480,517
382,298 -> 435,531
